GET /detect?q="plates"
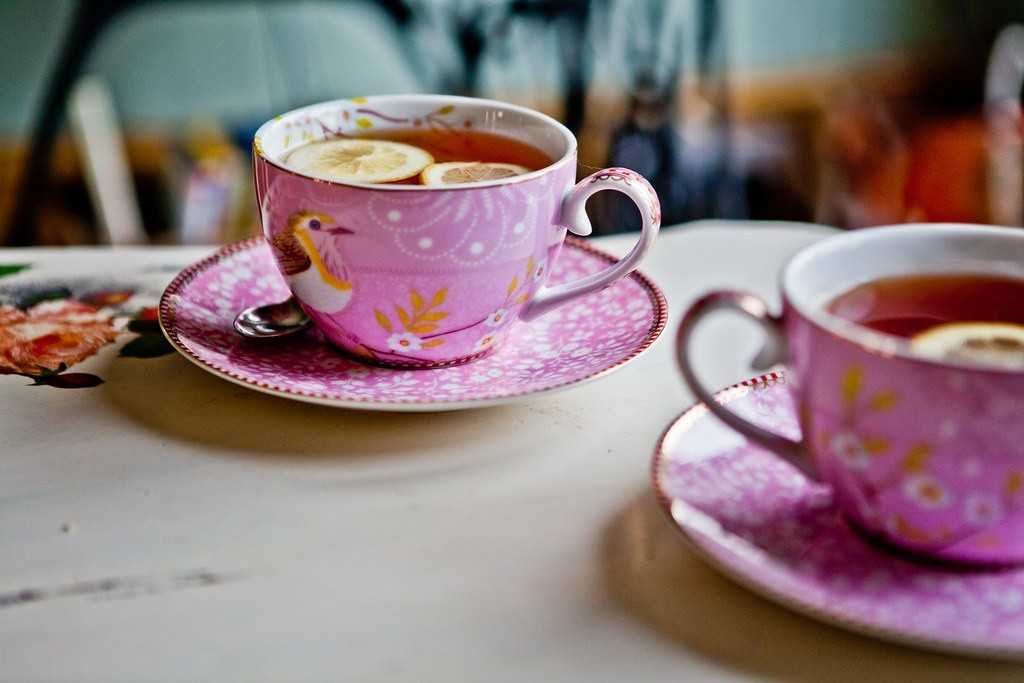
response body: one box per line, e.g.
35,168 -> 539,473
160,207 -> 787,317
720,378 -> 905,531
654,365 -> 1024,664
158,233 -> 669,414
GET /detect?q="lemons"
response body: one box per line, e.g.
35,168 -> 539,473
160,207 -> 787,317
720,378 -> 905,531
285,139 -> 435,183
905,320 -> 1024,359
420,161 -> 534,186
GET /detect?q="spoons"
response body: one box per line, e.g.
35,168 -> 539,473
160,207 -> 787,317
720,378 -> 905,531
234,295 -> 311,343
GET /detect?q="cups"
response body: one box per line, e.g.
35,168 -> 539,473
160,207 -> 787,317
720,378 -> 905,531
671,222 -> 1024,573
250,93 -> 665,370
